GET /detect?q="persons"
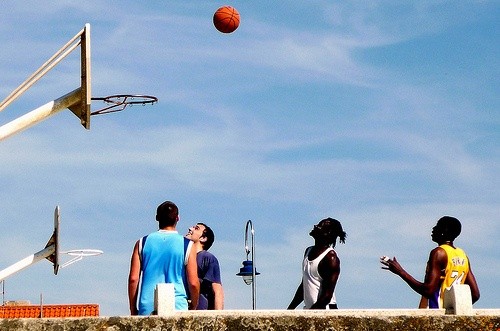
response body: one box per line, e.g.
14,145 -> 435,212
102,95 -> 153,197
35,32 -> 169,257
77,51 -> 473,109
380,216 -> 480,309
286,217 -> 348,310
184,222 -> 225,311
128,201 -> 200,315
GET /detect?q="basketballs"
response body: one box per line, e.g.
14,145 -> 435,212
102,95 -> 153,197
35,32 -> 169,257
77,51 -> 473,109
213,6 -> 240,34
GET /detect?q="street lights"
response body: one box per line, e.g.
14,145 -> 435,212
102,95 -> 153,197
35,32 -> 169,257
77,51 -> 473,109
236,220 -> 261,310
